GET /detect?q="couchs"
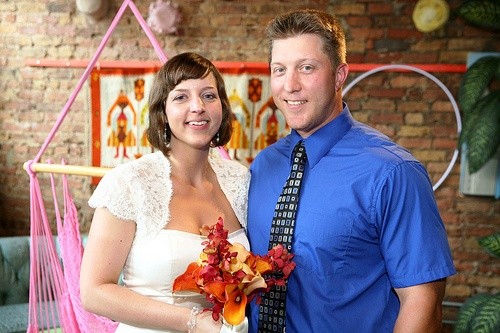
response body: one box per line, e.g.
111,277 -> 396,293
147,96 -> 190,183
0,237 -> 95,333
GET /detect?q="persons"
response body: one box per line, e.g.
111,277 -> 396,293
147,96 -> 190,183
247,10 -> 456,333
80,52 -> 251,333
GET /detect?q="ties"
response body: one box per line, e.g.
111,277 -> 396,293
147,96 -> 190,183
257,138 -> 308,333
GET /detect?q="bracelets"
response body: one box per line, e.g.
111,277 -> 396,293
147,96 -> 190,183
187,307 -> 199,333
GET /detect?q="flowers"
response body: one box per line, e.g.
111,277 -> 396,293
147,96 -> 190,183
172,217 -> 297,327
